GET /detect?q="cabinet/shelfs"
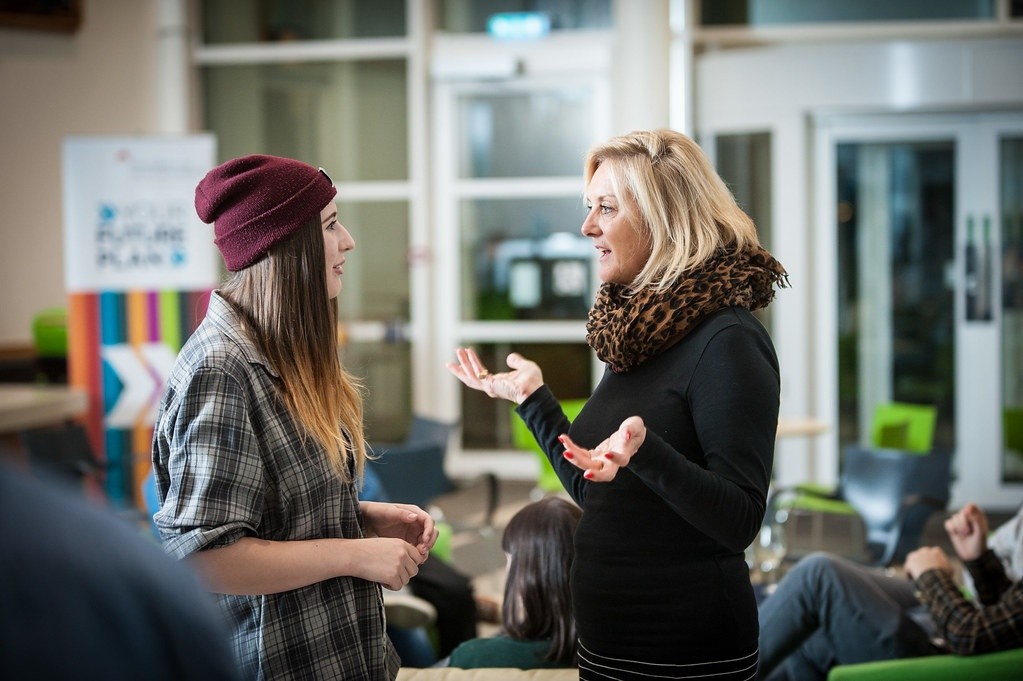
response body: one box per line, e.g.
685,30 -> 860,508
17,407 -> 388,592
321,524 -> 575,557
168,0 -> 692,484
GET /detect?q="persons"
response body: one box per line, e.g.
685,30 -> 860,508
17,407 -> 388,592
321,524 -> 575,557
755,504 -> 1023,681
363,504 -> 496,652
448,496 -> 581,668
151,154 -> 438,679
443,128 -> 781,680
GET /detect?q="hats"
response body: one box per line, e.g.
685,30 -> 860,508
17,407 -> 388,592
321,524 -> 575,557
193,150 -> 335,271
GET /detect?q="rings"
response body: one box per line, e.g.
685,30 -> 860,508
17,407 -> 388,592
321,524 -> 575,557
476,371 -> 490,379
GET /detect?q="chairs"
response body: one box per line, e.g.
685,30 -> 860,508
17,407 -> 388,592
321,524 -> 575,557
776,400 -> 936,566
